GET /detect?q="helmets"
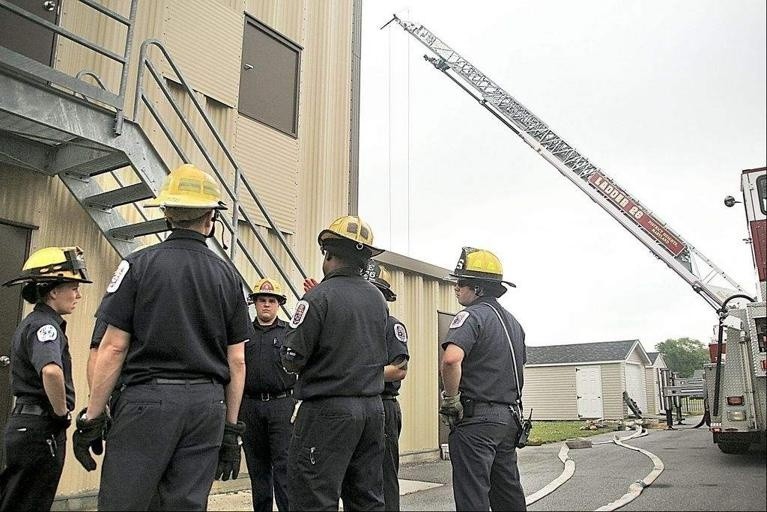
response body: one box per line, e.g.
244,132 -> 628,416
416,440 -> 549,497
359,259 -> 397,301
143,164 -> 229,209
248,279 -> 287,305
450,246 -> 517,288
318,215 -> 385,256
1,247 -> 93,287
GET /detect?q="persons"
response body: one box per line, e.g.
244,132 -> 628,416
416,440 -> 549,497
0,245 -> 94,511
437,245 -> 528,511
71,163 -> 256,511
85,318 -> 116,441
356,260 -> 410,511
246,275 -> 297,511
275,214 -> 391,511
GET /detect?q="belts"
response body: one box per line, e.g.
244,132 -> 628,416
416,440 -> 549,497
248,389 -> 293,401
10,400 -> 44,416
382,392 -> 398,400
147,374 -> 212,386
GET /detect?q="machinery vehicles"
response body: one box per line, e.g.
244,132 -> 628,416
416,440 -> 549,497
378,9 -> 767,457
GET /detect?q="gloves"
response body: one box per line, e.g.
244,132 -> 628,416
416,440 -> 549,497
55,410 -> 72,429
439,391 -> 464,429
72,406 -> 103,471
215,420 -> 247,481
